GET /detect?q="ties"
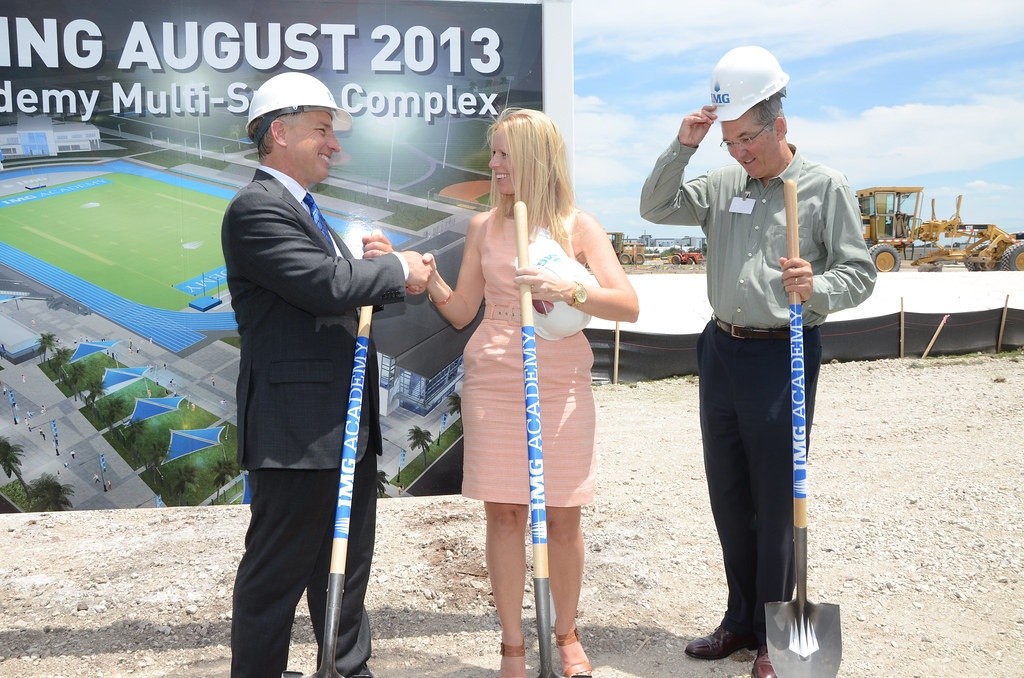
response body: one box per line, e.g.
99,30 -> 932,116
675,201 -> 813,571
302,192 -> 335,251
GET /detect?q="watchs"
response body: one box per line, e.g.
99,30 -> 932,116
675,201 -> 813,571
568,280 -> 587,307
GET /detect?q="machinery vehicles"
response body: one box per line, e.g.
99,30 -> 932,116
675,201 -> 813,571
854,186 -> 925,272
606,232 -> 645,264
671,253 -> 704,265
910,195 -> 1024,271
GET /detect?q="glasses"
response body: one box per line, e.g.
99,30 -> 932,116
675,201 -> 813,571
532,299 -> 553,315
719,119 -> 774,151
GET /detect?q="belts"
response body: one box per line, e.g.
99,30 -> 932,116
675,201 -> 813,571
483,304 -> 521,326
711,316 -> 790,341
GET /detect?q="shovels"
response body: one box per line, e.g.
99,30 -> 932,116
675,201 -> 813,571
512,200 -> 597,678
281,232 -> 394,678
765,183 -> 842,678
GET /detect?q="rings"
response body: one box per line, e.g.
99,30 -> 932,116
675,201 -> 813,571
531,285 -> 533,290
379,250 -> 381,256
795,278 -> 798,285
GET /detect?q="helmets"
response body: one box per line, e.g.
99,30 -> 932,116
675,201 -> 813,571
710,47 -> 790,121
245,72 -> 353,133
514,238 -> 592,341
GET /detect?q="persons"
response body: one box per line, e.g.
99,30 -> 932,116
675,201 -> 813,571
640,46 -> 878,678
407,107 -> 639,678
220,70 -> 432,678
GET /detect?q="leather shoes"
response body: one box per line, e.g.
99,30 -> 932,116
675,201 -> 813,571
685,626 -> 758,660
753,643 -> 776,678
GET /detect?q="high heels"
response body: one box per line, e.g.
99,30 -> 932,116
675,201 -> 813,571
499,638 -> 525,678
555,628 -> 591,678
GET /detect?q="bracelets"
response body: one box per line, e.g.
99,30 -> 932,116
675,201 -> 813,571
429,290 -> 453,306
